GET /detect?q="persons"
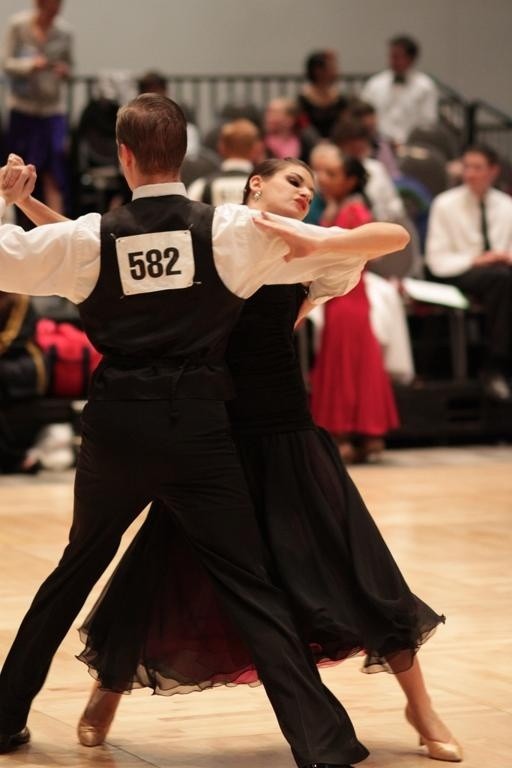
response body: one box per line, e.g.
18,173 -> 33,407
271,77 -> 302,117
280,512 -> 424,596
2,151 -> 465,763
0,0 -> 512,472
0,93 -> 371,768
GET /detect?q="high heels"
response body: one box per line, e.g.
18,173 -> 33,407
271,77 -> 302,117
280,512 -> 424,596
74,663 -> 120,749
402,687 -> 463,764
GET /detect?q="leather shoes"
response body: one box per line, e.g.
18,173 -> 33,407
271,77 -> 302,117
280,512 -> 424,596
1,725 -> 32,754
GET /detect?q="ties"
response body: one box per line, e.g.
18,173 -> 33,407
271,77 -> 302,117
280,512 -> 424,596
478,201 -> 492,252
